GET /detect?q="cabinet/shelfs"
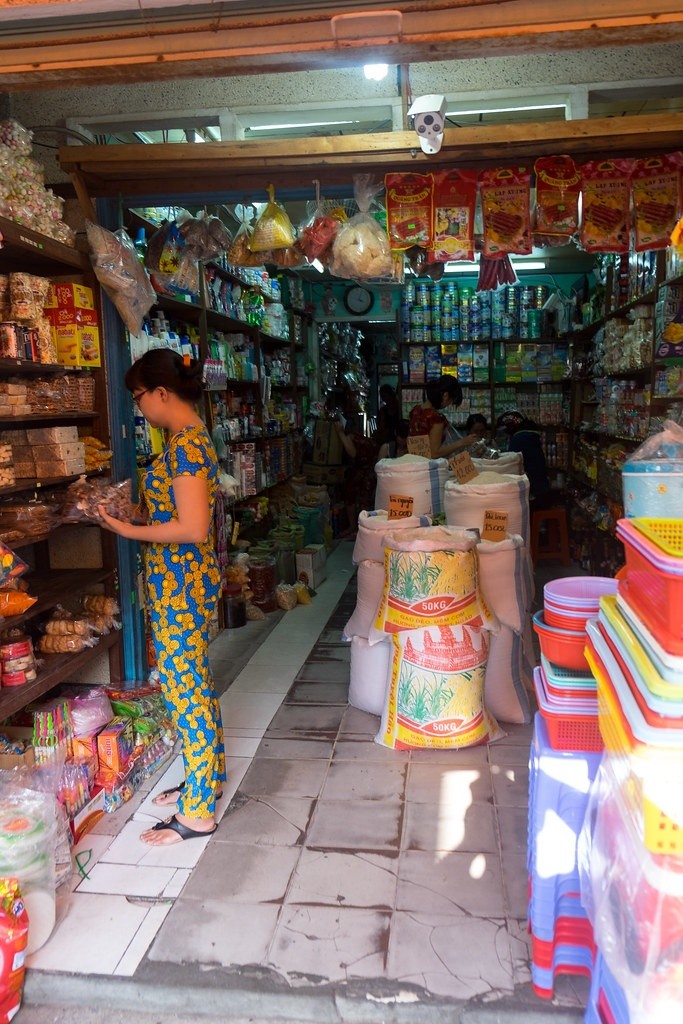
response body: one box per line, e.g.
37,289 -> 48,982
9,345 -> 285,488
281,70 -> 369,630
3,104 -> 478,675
573,250 -> 683,577
0,215 -> 126,724
125,207 -> 312,544
491,338 -> 574,529
398,341 -> 492,436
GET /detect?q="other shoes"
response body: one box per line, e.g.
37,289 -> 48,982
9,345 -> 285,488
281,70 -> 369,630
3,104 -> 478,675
339,530 -> 357,540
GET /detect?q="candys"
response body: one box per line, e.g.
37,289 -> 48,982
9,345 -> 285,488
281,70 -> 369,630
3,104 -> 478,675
0,117 -> 75,246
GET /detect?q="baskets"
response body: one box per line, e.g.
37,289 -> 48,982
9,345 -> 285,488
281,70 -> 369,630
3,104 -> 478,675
534,517 -> 683,961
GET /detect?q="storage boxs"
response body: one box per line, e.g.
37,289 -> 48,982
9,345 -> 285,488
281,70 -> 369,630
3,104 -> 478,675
0,725 -> 35,770
296,544 -> 327,589
400,343 -> 568,384
303,462 -> 345,484
55,308 -> 101,368
44,282 -> 94,310
236,442 -> 263,496
654,286 -> 683,363
73,727 -> 104,775
44,309 -> 57,353
97,715 -> 134,776
69,785 -> 106,846
312,420 -> 343,466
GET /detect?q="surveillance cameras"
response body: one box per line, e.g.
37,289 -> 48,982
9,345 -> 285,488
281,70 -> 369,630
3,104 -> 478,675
406,93 -> 448,139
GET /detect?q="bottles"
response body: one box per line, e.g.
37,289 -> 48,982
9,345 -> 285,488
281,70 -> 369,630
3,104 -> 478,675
134,228 -> 151,263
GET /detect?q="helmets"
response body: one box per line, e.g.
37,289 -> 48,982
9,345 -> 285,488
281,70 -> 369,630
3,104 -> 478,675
495,411 -> 525,430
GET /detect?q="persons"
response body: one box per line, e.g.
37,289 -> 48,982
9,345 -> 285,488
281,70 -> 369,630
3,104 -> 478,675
98,347 -> 227,845
329,375 -> 552,543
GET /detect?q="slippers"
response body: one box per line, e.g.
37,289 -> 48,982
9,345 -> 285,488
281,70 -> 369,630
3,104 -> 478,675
152,781 -> 223,807
140,814 -> 218,846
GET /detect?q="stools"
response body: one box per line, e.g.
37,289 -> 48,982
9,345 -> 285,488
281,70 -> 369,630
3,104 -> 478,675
524,711 -> 604,1001
583,948 -> 641,1024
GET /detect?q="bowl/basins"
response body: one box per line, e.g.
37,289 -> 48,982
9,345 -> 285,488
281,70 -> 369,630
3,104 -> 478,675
533,577 -> 620,672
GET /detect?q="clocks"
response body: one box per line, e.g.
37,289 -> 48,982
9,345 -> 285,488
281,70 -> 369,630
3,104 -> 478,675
342,284 -> 374,316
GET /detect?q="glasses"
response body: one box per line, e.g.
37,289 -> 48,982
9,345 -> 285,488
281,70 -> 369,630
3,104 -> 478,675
133,385 -> 174,408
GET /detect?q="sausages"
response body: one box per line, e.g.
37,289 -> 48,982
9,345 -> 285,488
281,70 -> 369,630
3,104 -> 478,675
542,202 -> 572,222
486,211 -> 521,234
585,203 -> 622,229
397,217 -> 423,238
476,253 -> 516,291
636,202 -> 675,225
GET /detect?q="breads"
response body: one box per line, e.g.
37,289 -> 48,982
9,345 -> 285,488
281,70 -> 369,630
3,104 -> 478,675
605,301 -> 652,369
39,592 -> 118,653
1,383 -> 85,487
